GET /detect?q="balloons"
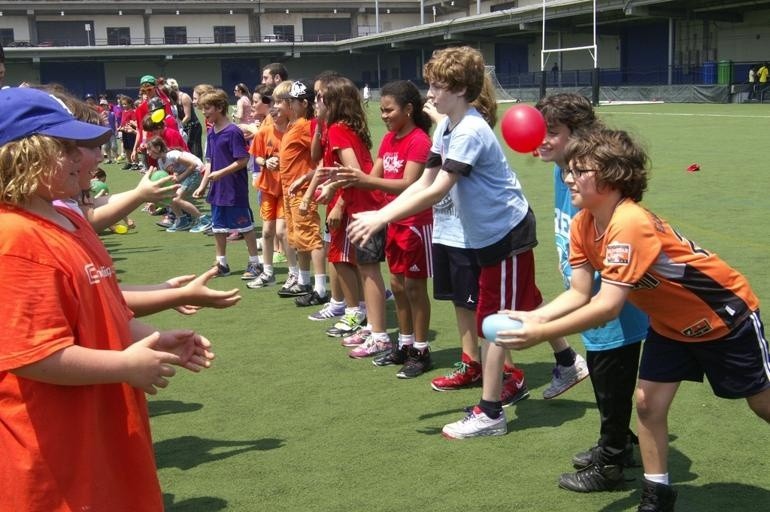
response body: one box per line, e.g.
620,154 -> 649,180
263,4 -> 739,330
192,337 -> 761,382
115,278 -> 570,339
502,105 -> 546,153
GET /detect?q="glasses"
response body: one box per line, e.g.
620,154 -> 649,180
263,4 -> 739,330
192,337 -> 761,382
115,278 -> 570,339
234,89 -> 238,91
560,167 -> 604,183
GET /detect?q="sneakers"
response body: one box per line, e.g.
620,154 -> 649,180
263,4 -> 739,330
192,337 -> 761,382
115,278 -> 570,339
247,271 -> 275,289
229,234 -> 243,240
633,478 -> 678,512
543,354 -> 590,399
156,214 -> 176,228
348,336 -> 393,360
208,262 -> 231,277
372,343 -> 413,367
342,328 -> 372,346
442,406 -> 508,439
278,281 -> 312,296
385,289 -> 393,300
307,302 -> 346,321
131,164 -> 139,170
104,160 -> 113,164
241,262 -> 264,279
121,163 -> 132,169
561,449 -> 625,491
431,352 -> 483,391
189,215 -> 213,233
574,432 -> 635,466
397,345 -> 435,379
166,215 -> 191,232
295,290 -> 329,306
151,208 -> 165,215
325,312 -> 366,336
282,272 -> 298,290
473,364 -> 530,415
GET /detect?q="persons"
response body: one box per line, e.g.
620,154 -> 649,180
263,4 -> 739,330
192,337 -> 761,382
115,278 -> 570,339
363,81 -> 373,111
312,80 -> 394,358
286,76 -> 368,322
493,127 -> 770,512
423,67 -> 531,408
144,135 -> 214,236
0,83 -> 243,316
142,116 -> 190,229
191,88 -> 263,280
129,74 -> 173,213
308,76 -> 392,324
315,81 -> 434,381
756,62 -> 770,100
247,82 -> 297,293
98,99 -> 120,163
536,92 -> 648,492
116,94 -> 139,171
345,47 -> 589,442
83,91 -> 104,114
84,167 -> 136,235
165,76 -> 204,163
133,88 -> 150,112
233,83 -> 256,141
146,100 -> 177,131
273,78 -> 331,308
1,87 -> 216,512
193,84 -> 245,240
159,76 -> 182,126
258,62 -> 289,249
250,102 -> 286,265
79,164 -> 182,235
747,64 -> 756,99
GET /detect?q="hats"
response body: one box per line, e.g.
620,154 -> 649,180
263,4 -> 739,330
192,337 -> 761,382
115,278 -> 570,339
83,93 -> 97,99
0,86 -> 113,145
140,75 -> 158,84
278,79 -> 315,101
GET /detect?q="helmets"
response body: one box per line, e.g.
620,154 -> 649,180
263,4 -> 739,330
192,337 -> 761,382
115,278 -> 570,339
147,97 -> 165,111
